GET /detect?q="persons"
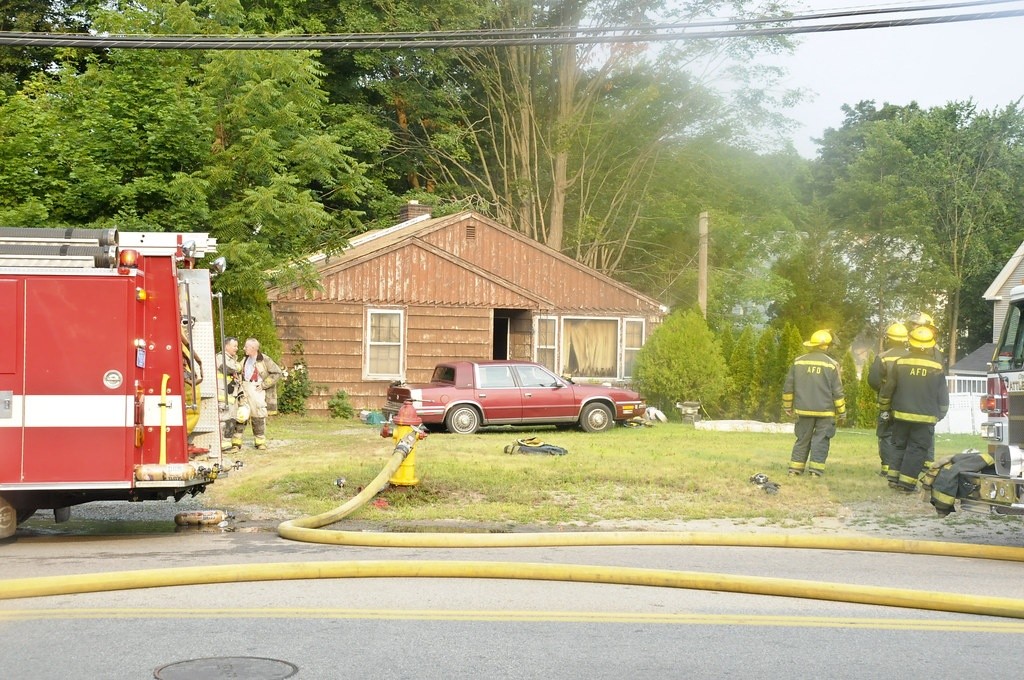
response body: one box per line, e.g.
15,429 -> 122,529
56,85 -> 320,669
214,336 -> 282,454
781,330 -> 847,482
867,311 -> 950,494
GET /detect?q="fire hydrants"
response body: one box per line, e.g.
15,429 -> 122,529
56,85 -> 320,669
379,398 -> 438,490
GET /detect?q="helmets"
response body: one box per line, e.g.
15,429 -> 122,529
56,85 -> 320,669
909,326 -> 936,349
803,330 -> 832,350
886,323 -> 909,343
909,311 -> 940,334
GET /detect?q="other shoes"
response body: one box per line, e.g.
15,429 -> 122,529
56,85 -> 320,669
257,444 -> 266,450
888,480 -> 903,488
231,446 -> 239,453
899,485 -> 922,494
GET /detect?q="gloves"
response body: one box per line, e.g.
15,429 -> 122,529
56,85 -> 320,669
836,411 -> 847,426
879,409 -> 890,423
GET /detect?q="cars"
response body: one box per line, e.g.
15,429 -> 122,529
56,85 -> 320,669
385,357 -> 649,435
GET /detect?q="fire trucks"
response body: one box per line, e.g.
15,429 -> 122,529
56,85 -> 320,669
956,278 -> 1024,517
0,225 -> 242,540
